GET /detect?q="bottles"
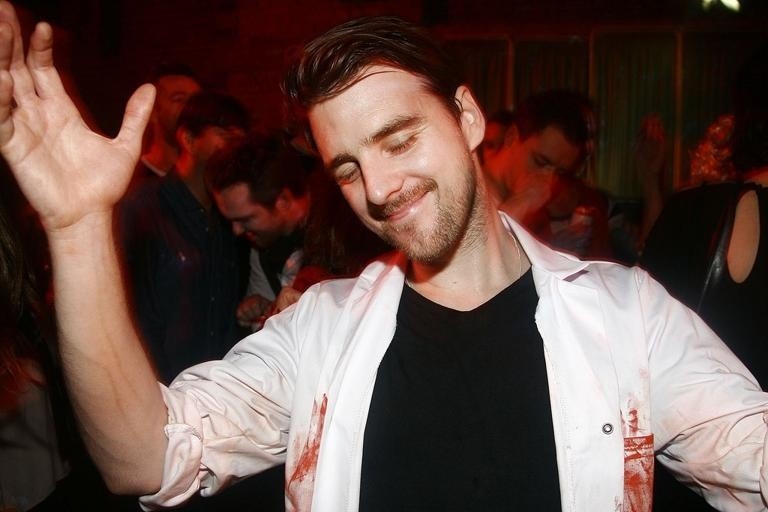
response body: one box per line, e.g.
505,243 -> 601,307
567,205 -> 596,262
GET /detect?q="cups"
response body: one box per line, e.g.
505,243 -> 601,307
251,282 -> 301,333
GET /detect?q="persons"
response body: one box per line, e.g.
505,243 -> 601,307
2,1 -> 768,511
2,66 -> 767,512
